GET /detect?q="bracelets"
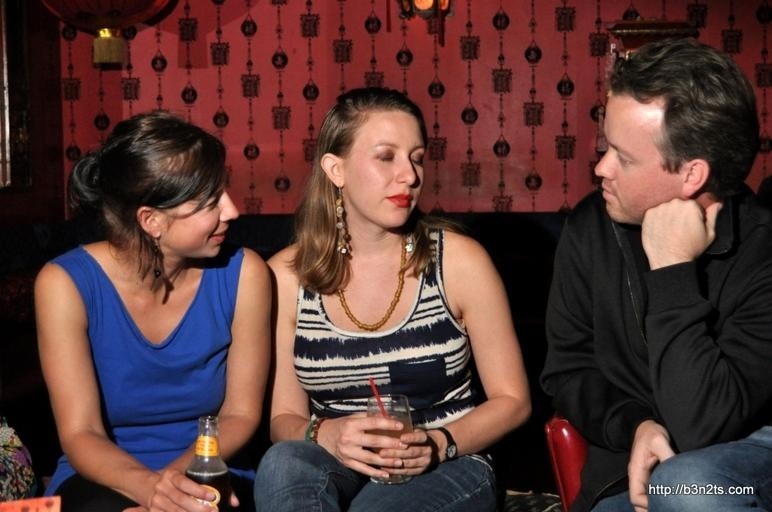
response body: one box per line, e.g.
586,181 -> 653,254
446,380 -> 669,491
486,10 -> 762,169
305,417 -> 330,444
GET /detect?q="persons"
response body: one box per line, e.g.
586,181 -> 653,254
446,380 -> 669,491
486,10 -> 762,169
539,37 -> 772,512
33,108 -> 271,512
253,84 -> 532,512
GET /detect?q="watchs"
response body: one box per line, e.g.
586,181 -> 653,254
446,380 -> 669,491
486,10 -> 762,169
437,427 -> 456,463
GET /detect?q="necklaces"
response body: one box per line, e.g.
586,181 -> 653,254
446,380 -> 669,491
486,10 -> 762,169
337,233 -> 406,331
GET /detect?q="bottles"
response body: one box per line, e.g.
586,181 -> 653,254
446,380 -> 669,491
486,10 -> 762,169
183,414 -> 233,512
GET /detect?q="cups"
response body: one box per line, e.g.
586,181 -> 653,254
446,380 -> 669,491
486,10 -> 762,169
364,394 -> 416,486
